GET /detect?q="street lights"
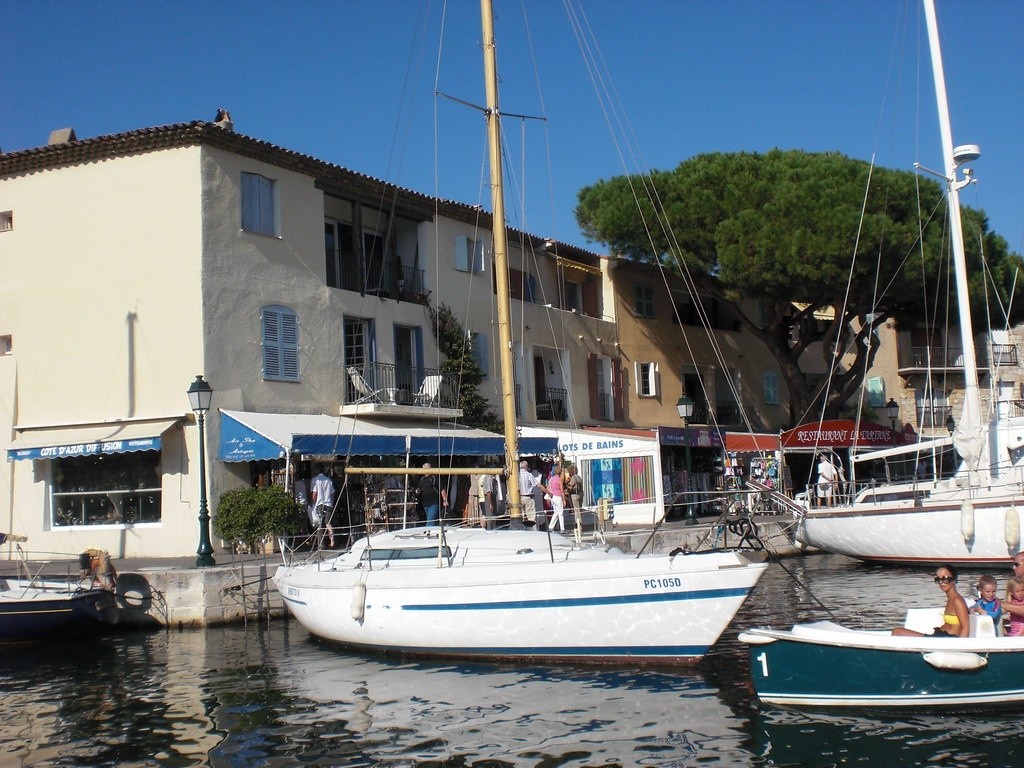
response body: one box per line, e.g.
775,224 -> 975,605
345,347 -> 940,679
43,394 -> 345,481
946,415 -> 956,473
883,398 -> 902,482
186,375 -> 217,567
677,392 -> 700,525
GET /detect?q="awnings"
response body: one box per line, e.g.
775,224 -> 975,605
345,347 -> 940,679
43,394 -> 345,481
217,407 -> 560,463
4,416 -> 185,460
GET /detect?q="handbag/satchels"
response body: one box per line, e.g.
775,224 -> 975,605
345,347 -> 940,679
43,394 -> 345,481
570,475 -> 580,494
307,505 -> 322,530
543,478 -> 552,500
820,482 -> 830,491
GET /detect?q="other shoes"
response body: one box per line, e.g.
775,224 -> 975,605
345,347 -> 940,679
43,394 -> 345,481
327,543 -> 336,549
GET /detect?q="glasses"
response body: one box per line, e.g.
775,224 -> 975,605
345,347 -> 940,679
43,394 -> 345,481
933,576 -> 954,585
1013,562 -> 1024,568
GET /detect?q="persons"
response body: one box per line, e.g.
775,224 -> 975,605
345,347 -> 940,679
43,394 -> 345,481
530,465 -> 546,527
967,575 -> 1004,637
518,462 -> 554,531
891,564 -> 972,637
818,457 -> 838,507
997,549 -> 1024,615
309,466 -> 337,550
468,460 -> 508,531
564,465 -> 585,536
415,463 -> 450,527
998,576 -> 1023,640
292,471 -> 313,543
545,466 -> 569,535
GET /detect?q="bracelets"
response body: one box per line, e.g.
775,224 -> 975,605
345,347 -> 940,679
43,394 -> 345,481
443,499 -> 447,501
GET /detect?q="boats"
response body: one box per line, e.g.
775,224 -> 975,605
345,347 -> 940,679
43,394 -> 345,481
737,605 -> 1023,710
1,535 -> 124,646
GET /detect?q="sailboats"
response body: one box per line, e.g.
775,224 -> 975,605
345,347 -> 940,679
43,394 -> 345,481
269,42 -> 773,665
778,0 -> 1024,569
